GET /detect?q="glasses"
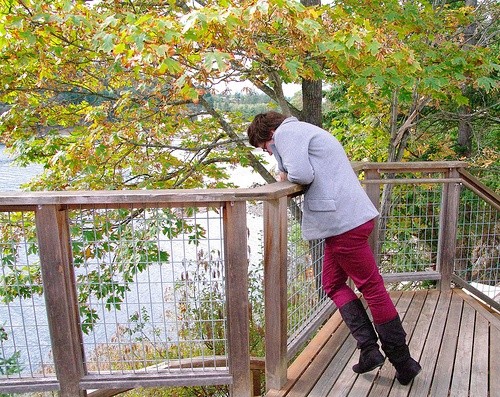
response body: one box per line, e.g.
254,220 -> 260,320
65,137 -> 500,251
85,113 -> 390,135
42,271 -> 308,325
263,140 -> 268,152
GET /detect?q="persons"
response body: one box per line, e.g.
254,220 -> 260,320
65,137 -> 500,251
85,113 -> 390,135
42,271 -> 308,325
248,111 -> 423,386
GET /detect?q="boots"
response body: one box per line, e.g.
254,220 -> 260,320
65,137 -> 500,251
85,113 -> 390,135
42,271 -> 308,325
374,313 -> 422,385
338,298 -> 385,374
